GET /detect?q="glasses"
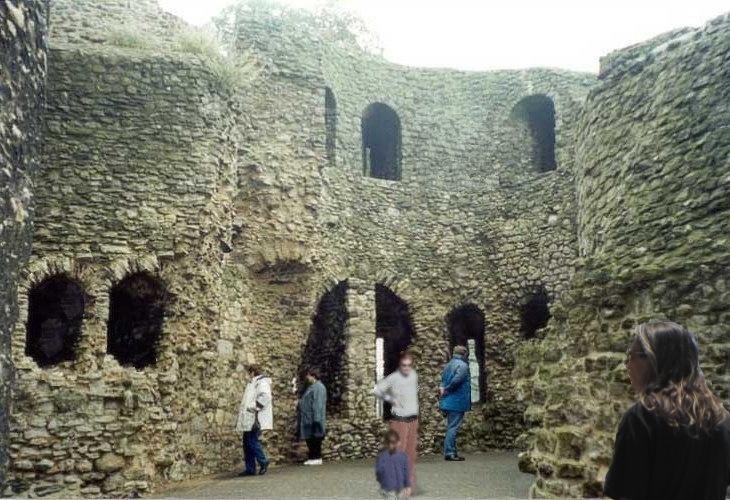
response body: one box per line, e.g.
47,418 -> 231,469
624,348 -> 644,362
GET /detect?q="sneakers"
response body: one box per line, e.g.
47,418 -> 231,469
303,458 -> 323,465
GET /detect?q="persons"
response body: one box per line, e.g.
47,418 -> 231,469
374,429 -> 411,500
299,366 -> 327,465
436,345 -> 471,461
236,363 -> 274,475
372,352 -> 419,489
600,320 -> 730,500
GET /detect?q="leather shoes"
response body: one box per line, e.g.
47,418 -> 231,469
445,454 -> 465,461
259,464 -> 267,474
239,471 -> 248,476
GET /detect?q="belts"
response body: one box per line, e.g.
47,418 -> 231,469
390,415 -> 417,422
